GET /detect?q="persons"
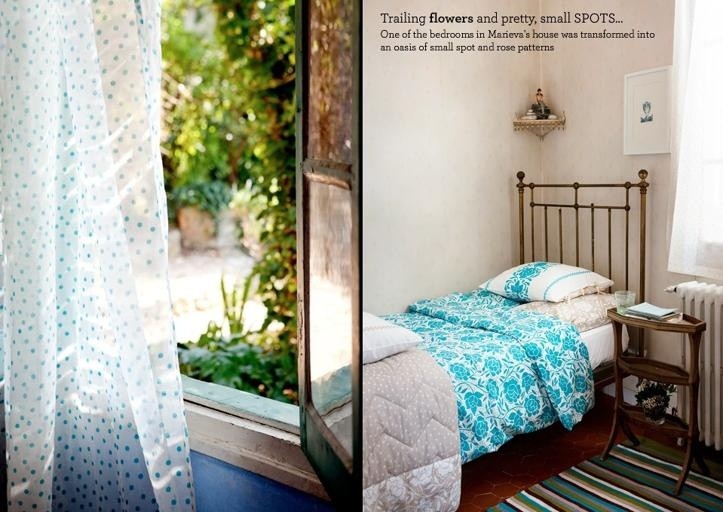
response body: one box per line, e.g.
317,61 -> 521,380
536,88 -> 544,102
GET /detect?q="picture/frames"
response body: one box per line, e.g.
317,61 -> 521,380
620,66 -> 672,156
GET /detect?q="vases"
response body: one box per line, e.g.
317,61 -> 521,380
645,411 -> 664,425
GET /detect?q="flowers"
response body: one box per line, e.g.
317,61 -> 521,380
633,380 -> 673,421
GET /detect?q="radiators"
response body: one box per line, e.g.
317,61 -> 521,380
664,278 -> 723,452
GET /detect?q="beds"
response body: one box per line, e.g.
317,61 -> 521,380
363,168 -> 651,510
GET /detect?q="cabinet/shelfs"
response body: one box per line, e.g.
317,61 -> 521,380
600,304 -> 710,497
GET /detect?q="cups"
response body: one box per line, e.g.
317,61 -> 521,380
613,290 -> 634,316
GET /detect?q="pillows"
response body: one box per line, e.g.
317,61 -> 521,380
362,311 -> 423,365
478,249 -> 621,337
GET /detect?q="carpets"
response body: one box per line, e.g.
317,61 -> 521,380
484,433 -> 723,512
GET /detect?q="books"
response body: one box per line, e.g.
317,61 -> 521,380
627,302 -> 680,321
